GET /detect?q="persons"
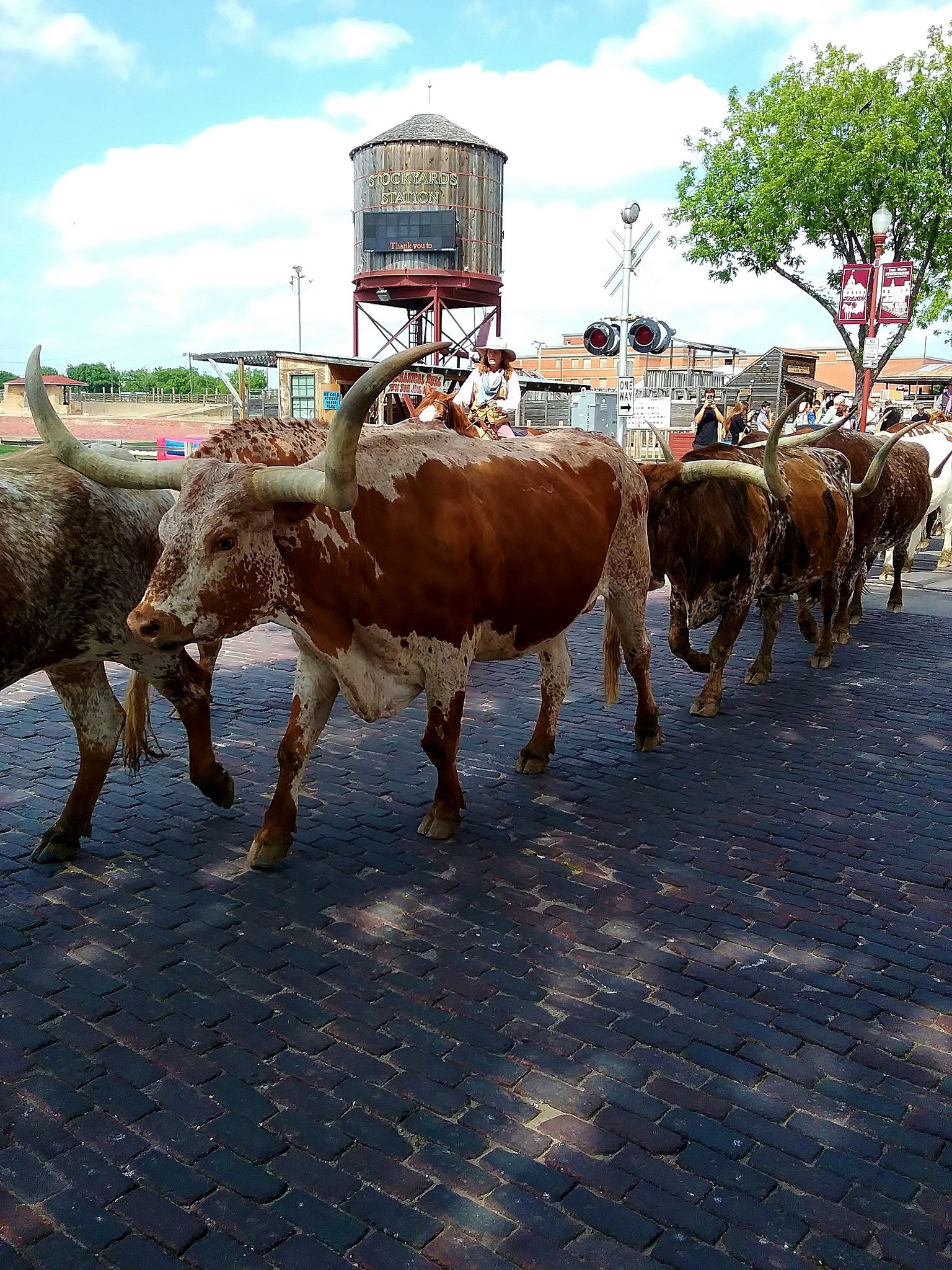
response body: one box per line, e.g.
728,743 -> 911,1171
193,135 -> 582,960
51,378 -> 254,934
724,400 -> 753,444
693,388 -> 724,450
451,336 -> 521,439
791,378 -> 952,432
757,401 -> 775,434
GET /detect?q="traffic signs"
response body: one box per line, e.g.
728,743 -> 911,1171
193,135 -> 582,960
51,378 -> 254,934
617,375 -> 636,419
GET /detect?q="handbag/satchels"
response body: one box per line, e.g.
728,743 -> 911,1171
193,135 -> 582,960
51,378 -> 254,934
738,426 -> 747,443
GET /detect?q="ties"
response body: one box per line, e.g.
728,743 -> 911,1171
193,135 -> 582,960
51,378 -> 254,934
944,389 -> 952,417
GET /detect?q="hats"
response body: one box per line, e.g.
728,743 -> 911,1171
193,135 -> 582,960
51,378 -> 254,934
476,337 -> 516,362
917,404 -> 925,409
799,402 -> 806,413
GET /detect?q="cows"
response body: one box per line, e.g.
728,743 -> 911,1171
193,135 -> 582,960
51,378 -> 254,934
25,341 -> 660,870
740,407 -> 952,644
637,391 -> 854,717
0,441 -> 235,864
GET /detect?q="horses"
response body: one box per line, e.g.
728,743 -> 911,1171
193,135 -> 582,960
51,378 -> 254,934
408,383 -> 549,438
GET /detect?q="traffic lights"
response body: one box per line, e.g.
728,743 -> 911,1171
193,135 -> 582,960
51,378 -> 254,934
585,314 -> 660,357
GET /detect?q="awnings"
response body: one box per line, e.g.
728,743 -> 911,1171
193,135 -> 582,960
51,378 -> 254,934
783,376 -> 849,394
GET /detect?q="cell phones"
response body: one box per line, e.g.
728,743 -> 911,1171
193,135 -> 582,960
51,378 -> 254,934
707,395 -> 712,405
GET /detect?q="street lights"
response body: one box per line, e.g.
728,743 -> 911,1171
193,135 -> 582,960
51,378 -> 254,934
182,352 -> 193,394
860,202 -> 893,432
289,265 -> 312,353
